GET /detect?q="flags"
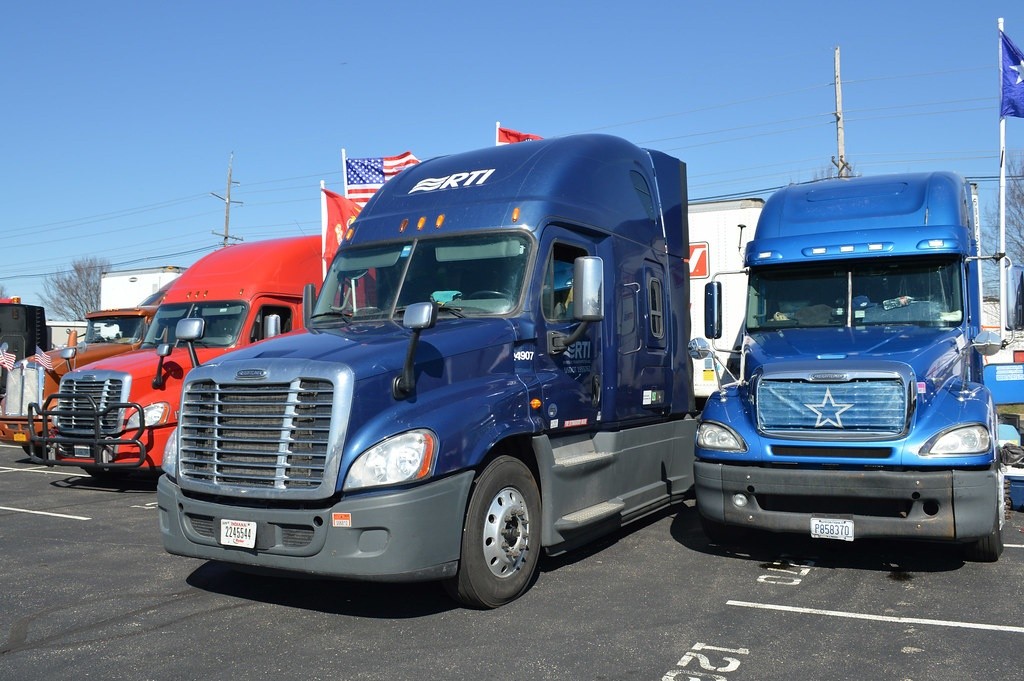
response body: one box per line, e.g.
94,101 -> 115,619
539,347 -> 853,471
496,127 -> 543,145
344,151 -> 420,209
999,29 -> 1024,119
36,346 -> 52,370
0,351 -> 16,371
322,190 -> 364,272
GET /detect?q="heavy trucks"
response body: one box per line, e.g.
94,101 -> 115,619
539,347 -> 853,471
157,133 -> 765,607
0,276 -> 180,458
688,171 -> 1015,562
29,234 -> 322,484
